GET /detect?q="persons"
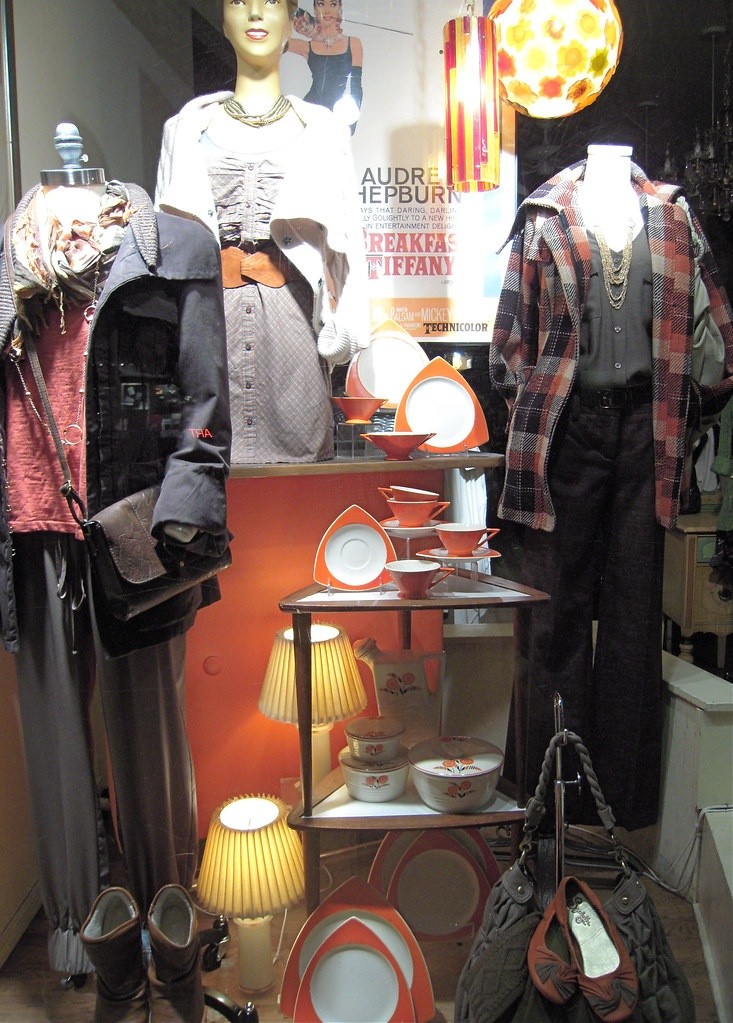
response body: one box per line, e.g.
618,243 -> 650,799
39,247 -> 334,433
0,167 -> 233,986
489,142 -> 732,831
154,0 -> 371,465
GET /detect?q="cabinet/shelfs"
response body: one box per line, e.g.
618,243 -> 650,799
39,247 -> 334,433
278,557 -> 550,921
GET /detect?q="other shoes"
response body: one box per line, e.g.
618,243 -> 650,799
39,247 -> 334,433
527,896 -> 576,1004
554,876 -> 640,1023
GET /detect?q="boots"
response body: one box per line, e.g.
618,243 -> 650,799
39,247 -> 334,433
80,887 -> 151,1023
148,884 -> 205,1023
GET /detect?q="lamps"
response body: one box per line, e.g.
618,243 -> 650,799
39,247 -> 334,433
259,621 -> 367,795
196,793 -> 305,995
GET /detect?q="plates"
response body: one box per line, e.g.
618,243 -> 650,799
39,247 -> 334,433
394,356 -> 489,454
367,826 -> 504,947
416,546 -> 501,563
312,504 -> 398,591
277,874 -> 435,1023
346,319 -> 431,410
379,517 -> 450,538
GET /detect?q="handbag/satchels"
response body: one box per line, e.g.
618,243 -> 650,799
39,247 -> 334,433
454,731 -> 695,1023
82,481 -> 232,622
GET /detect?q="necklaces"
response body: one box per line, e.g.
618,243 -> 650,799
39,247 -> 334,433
224,92 -> 290,127
580,177 -> 631,309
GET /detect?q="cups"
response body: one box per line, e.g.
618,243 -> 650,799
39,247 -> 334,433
359,432 -> 436,461
330,395 -> 389,424
378,485 -> 450,526
385,560 -> 455,600
436,524 -> 500,556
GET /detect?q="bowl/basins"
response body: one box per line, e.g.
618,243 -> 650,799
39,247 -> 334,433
347,715 -> 405,763
406,736 -> 505,814
338,747 -> 410,803
